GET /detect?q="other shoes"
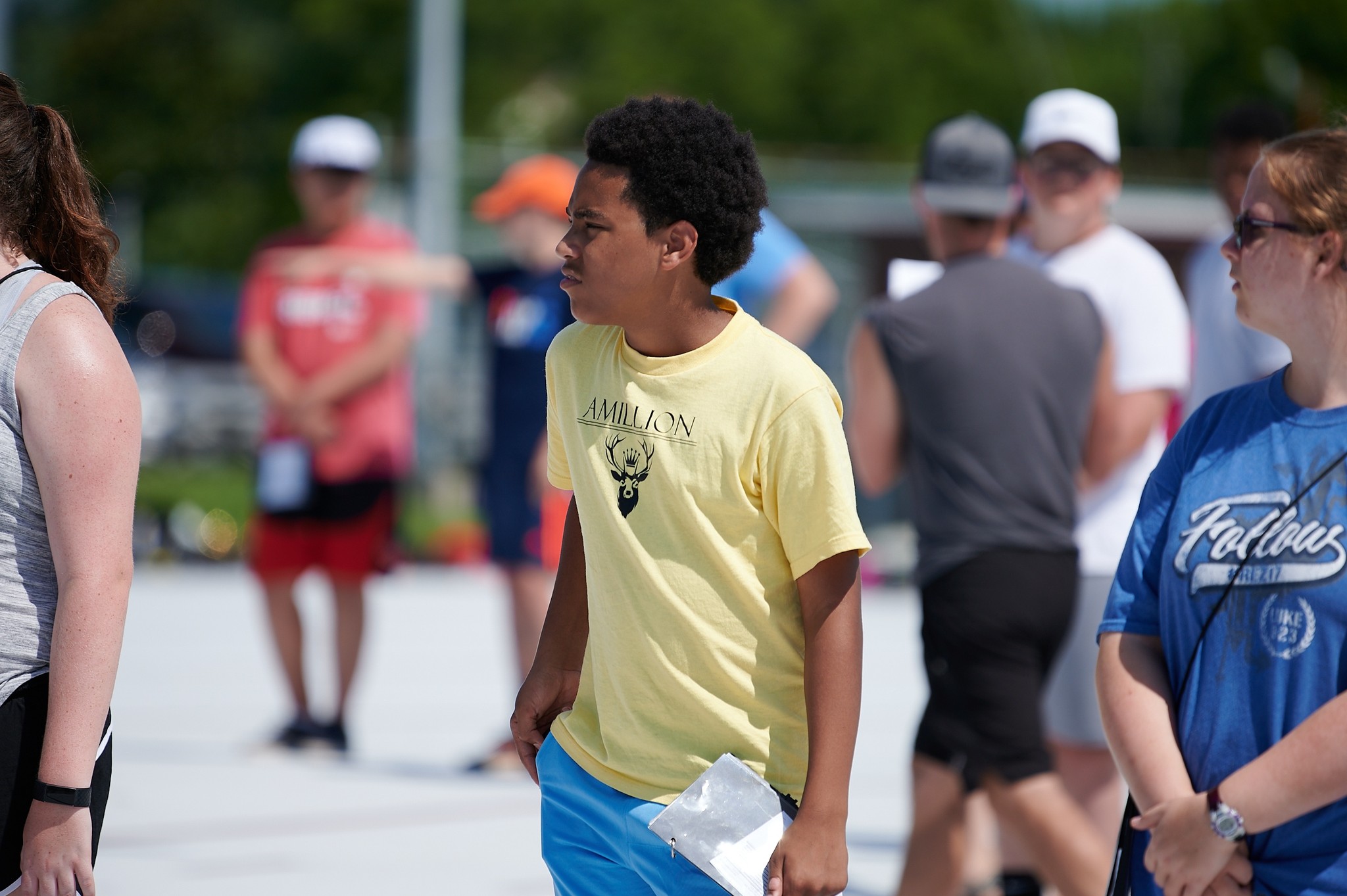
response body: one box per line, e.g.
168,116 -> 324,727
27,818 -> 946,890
323,721 -> 352,751
283,714 -> 317,747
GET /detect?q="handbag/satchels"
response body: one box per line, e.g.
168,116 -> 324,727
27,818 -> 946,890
1105,794 -> 1136,896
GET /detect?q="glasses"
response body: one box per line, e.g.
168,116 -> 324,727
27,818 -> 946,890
1235,210 -> 1328,251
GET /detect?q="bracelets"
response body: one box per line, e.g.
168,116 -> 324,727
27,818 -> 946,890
30,781 -> 91,807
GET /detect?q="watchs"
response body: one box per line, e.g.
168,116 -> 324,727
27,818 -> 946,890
1206,781 -> 1249,846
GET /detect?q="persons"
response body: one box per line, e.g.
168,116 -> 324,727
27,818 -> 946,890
0,69 -> 141,894
1175,110 -> 1293,416
841,113 -> 1130,895
999,87 -> 1191,895
705,204 -> 838,350
240,114 -> 425,755
1097,130 -> 1346,895
508,94 -> 872,896
471,151 -> 577,768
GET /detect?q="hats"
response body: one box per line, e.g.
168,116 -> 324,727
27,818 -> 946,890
292,114 -> 381,173
475,155 -> 580,220
1017,90 -> 1121,165
919,115 -> 1013,218
